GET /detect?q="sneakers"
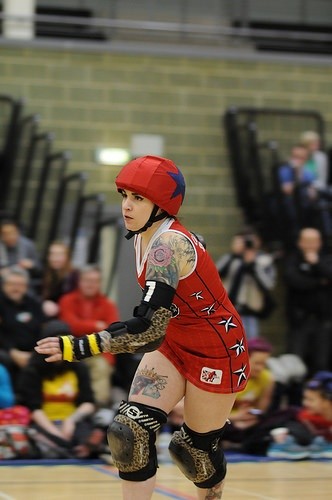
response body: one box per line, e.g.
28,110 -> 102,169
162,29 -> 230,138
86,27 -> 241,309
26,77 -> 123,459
267,437 -> 310,460
306,440 -> 331,459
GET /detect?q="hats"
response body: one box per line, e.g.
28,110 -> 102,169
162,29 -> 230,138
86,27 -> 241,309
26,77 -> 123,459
307,372 -> 332,400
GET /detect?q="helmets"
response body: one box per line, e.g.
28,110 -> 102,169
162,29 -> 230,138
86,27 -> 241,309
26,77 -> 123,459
115,156 -> 185,216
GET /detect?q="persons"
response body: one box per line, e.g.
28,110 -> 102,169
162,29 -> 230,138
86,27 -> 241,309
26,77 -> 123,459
215,226 -> 332,459
34,155 -> 251,500
0,219 -> 119,412
277,130 -> 329,200
